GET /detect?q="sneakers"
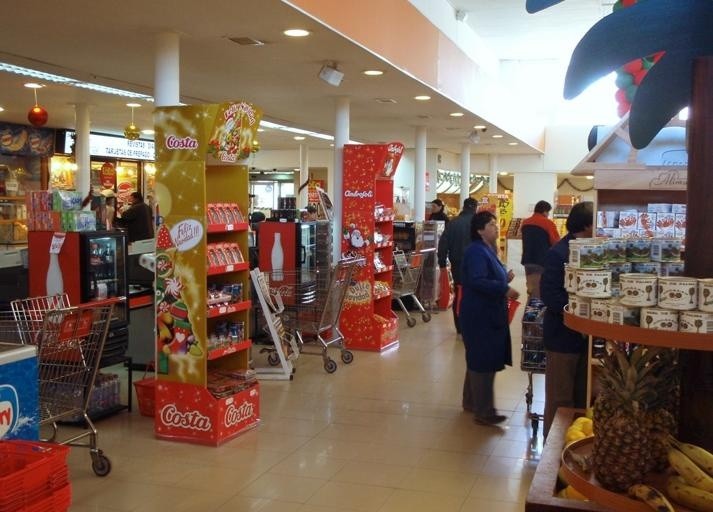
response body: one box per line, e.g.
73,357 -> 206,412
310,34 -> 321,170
474,415 -> 505,424
463,408 -> 496,415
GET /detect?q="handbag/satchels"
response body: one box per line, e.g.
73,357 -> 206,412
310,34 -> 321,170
439,270 -> 455,308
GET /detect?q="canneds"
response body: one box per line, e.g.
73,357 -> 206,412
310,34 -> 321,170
639,306 -> 679,331
657,276 -> 698,310
618,272 -> 658,308
679,309 -> 713,334
697,277 -> 713,314
627,237 -> 684,275
560,237 -> 624,324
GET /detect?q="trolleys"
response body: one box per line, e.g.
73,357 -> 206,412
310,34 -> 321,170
249,256 -> 366,373
392,246 -> 437,328
521,298 -> 545,438
0,292 -> 126,478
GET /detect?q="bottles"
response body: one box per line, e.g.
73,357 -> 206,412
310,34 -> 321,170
270,231 -> 285,281
46,252 -> 63,322
91,281 -> 114,301
92,241 -> 114,281
51,370 -> 120,422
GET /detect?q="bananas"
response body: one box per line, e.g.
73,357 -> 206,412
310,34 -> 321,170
625,430 -> 712,512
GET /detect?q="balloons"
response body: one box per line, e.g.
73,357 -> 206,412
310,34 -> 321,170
124,125 -> 142,141
251,140 -> 261,154
609,0 -> 666,118
27,106 -> 49,128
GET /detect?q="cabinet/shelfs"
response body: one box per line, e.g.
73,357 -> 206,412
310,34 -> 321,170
341,143 -> 404,352
155,101 -> 264,447
525,50 -> 713,512
571,111 -> 688,408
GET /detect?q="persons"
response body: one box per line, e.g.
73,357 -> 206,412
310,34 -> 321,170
429,198 -> 449,232
249,193 -> 255,209
438,197 -> 478,333
115,192 -> 154,240
537,201 -> 594,447
305,207 -> 317,219
247,211 -> 265,232
82,191 -> 96,212
458,211 -> 520,425
521,200 -> 560,343
105,196 -> 122,232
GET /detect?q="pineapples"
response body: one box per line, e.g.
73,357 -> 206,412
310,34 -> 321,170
590,337 -> 678,492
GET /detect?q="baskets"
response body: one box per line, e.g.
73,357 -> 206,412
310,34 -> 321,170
456,285 -> 520,324
0,439 -> 72,512
132,376 -> 155,416
